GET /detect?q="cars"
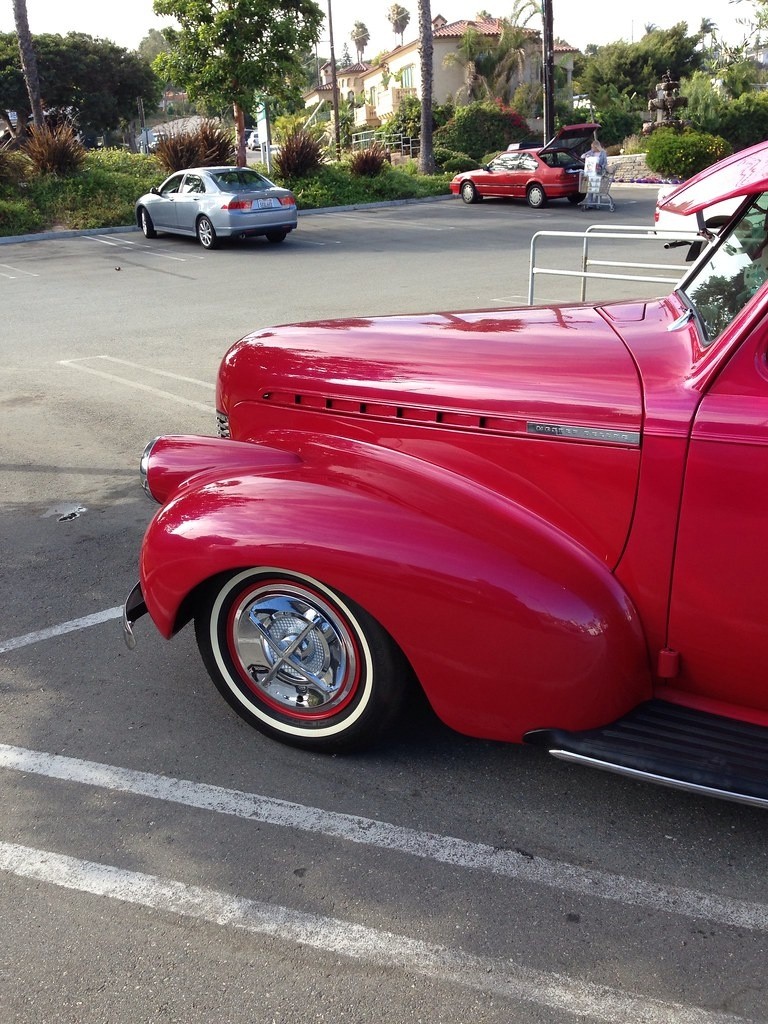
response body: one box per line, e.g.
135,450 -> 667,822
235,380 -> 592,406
137,166 -> 298,251
118,144 -> 767,758
648,180 -> 753,241
449,122 -> 600,207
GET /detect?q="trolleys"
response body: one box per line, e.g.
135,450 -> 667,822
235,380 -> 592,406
575,164 -> 617,213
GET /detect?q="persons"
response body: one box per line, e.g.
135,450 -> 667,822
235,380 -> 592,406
581,141 -> 607,210
